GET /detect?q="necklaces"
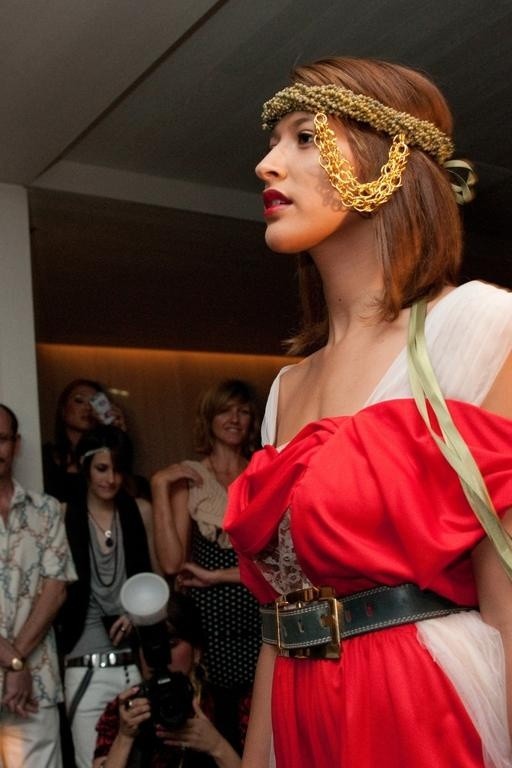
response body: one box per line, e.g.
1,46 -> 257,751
86,504 -> 116,547
87,524 -> 118,588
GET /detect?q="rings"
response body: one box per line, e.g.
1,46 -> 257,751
120,627 -> 127,633
128,701 -> 133,708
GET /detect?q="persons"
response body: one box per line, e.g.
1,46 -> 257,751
224,55 -> 512,768
42,377 -> 133,477
92,589 -> 250,768
150,374 -> 263,697
0,404 -> 80,768
44,419 -> 166,768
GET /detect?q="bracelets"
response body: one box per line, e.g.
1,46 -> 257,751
3,656 -> 25,673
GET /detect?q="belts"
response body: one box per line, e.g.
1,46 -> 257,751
256,583 -> 479,663
60,651 -> 139,675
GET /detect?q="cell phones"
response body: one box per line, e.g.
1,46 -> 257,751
89,392 -> 116,425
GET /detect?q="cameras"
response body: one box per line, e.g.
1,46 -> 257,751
121,572 -> 194,731
